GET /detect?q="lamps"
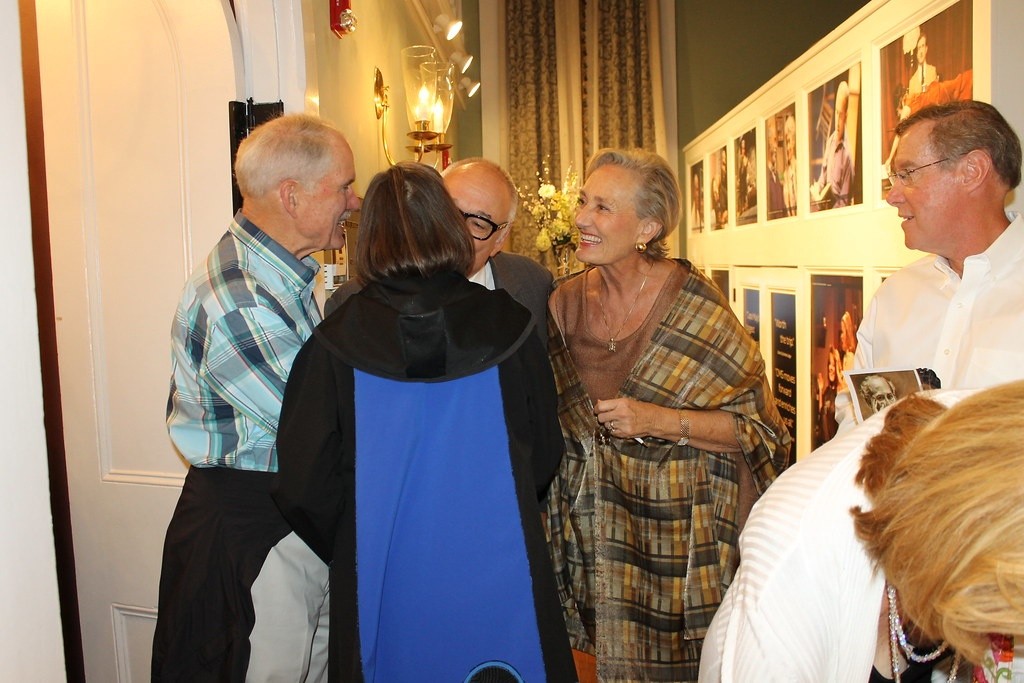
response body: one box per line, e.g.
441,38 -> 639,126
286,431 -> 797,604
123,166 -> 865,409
432,14 -> 463,41
450,52 -> 473,74
459,77 -> 481,98
374,45 -> 457,170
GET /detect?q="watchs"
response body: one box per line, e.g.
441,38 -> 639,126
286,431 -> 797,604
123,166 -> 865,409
676,409 -> 690,447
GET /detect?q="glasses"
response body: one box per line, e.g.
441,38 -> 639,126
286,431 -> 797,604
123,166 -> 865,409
887,152 -> 968,186
459,208 -> 508,241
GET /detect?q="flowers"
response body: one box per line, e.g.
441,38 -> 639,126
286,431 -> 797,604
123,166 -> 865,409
514,154 -> 582,278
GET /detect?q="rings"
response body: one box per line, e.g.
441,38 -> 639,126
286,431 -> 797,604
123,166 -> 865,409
608,421 -> 613,430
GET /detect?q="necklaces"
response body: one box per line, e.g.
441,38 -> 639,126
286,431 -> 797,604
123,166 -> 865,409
885,583 -> 963,683
598,257 -> 655,353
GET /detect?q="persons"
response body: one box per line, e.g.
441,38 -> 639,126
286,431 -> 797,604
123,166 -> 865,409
279,156 -> 580,683
833,100 -> 1024,429
147,117 -> 360,683
700,384 -> 1023,683
546,144 -> 789,683
